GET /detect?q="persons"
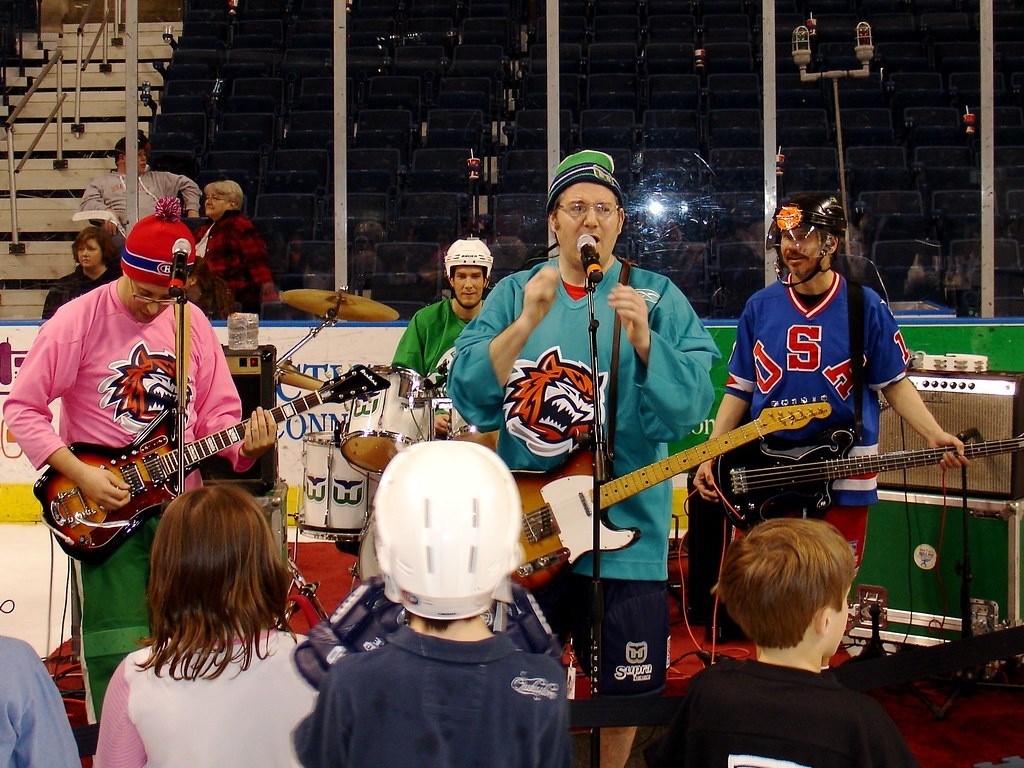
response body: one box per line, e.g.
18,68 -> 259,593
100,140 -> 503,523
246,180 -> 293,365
181,256 -> 226,322
350,206 -> 531,308
1,632 -> 82,768
718,200 -> 765,320
292,440 -> 570,768
89,485 -> 321,768
447,148 -> 720,768
391,238 -> 495,440
190,180 -> 278,318
623,518 -> 921,768
79,135 -> 202,244
692,192 -> 973,668
1,213 -> 278,767
42,226 -> 123,321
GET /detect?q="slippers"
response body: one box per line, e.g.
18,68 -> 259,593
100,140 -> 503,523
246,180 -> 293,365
558,202 -> 618,218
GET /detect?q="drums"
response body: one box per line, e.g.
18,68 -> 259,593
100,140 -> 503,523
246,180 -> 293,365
297,430 -> 384,537
448,395 -> 475,440
357,507 -> 382,582
339,364 -> 437,475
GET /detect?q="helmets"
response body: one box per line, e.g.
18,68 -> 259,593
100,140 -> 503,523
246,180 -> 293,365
373,439 -> 526,621
772,193 -> 848,238
444,237 -> 493,279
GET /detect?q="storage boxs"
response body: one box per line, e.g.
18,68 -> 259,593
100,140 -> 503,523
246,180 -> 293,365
842,488 -> 1024,647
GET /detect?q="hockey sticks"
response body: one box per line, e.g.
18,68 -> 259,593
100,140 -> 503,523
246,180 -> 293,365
72,209 -> 128,240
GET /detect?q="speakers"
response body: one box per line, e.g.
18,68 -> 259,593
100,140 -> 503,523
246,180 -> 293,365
199,344 -> 277,496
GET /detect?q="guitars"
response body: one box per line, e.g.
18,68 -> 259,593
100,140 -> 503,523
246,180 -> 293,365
715,430 -> 1024,531
32,364 -> 392,566
508,395 -> 833,588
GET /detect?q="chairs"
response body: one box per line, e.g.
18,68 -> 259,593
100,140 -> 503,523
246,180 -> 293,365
0,0 -> 42,128
150,0 -> 1024,320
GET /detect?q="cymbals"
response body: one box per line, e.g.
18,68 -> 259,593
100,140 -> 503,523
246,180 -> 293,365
274,363 -> 324,394
279,288 -> 398,323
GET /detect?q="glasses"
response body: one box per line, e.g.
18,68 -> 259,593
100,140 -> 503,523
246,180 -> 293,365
128,279 -> 180,307
204,195 -> 224,201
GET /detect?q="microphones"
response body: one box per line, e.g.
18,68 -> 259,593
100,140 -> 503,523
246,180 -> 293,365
576,234 -> 604,283
168,238 -> 191,298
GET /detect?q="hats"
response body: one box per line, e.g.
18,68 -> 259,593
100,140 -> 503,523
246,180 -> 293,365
547,150 -> 624,213
121,198 -> 195,286
